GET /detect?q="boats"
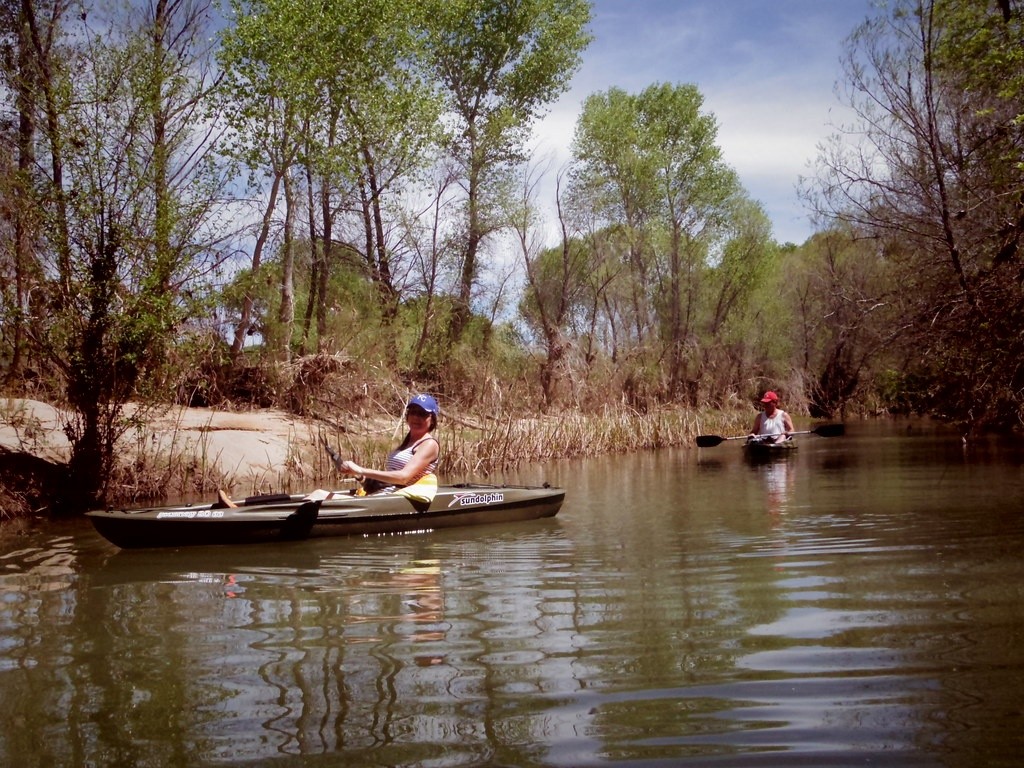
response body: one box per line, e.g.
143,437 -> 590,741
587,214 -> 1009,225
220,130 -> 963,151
82,478 -> 568,550
745,436 -> 798,462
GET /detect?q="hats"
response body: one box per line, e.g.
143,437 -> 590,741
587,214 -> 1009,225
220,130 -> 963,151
761,391 -> 778,403
405,393 -> 438,414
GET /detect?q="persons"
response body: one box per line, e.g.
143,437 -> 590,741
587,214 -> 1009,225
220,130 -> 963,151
748,391 -> 794,444
340,393 -> 439,499
